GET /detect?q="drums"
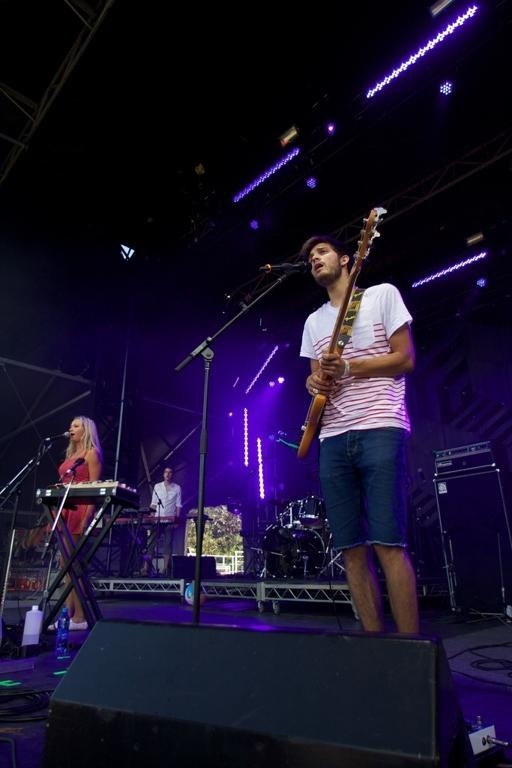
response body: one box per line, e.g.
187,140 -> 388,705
263,523 -> 326,580
298,495 -> 327,529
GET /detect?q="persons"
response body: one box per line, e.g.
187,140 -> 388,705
299,235 -> 421,639
45,414 -> 106,631
132,465 -> 184,578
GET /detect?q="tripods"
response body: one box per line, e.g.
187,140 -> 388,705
260,560 -> 276,578
319,532 -> 346,577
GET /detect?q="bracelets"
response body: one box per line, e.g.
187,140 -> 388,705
340,358 -> 350,379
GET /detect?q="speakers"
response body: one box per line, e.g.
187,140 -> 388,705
43,616 -> 478,768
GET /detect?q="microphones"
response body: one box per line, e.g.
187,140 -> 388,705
45,431 -> 70,442
63,457 -> 85,476
259,261 -> 312,274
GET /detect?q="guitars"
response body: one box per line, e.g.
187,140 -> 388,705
297,207 -> 387,461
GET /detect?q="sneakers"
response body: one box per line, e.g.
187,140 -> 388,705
48,616 -> 88,631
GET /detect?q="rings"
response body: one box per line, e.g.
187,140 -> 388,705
311,387 -> 319,394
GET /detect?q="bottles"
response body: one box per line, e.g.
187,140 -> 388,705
54,608 -> 70,655
22,605 -> 42,646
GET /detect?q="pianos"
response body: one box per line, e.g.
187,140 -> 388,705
106,515 -> 178,524
